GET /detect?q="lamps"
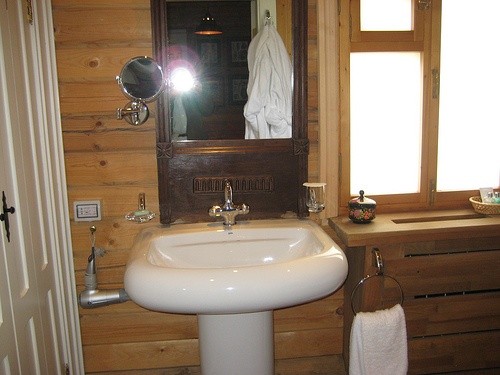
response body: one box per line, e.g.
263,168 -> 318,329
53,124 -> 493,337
193,3 -> 225,37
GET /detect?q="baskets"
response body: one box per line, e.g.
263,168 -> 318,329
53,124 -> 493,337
469,195 -> 500,215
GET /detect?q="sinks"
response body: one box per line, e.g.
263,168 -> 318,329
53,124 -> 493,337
122,225 -> 350,315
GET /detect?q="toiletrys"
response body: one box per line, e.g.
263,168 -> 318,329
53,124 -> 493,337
479,186 -> 500,204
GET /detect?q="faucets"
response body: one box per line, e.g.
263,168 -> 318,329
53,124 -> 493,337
222,179 -> 237,226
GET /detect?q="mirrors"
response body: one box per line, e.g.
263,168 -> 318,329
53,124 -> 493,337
149,0 -> 310,154
116,57 -> 164,126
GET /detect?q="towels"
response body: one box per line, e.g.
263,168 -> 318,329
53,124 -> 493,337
348,303 -> 408,375
243,19 -> 294,139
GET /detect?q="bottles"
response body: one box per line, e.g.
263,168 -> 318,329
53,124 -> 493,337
348,190 -> 377,223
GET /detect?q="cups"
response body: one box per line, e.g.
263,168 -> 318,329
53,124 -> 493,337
303,182 -> 327,212
